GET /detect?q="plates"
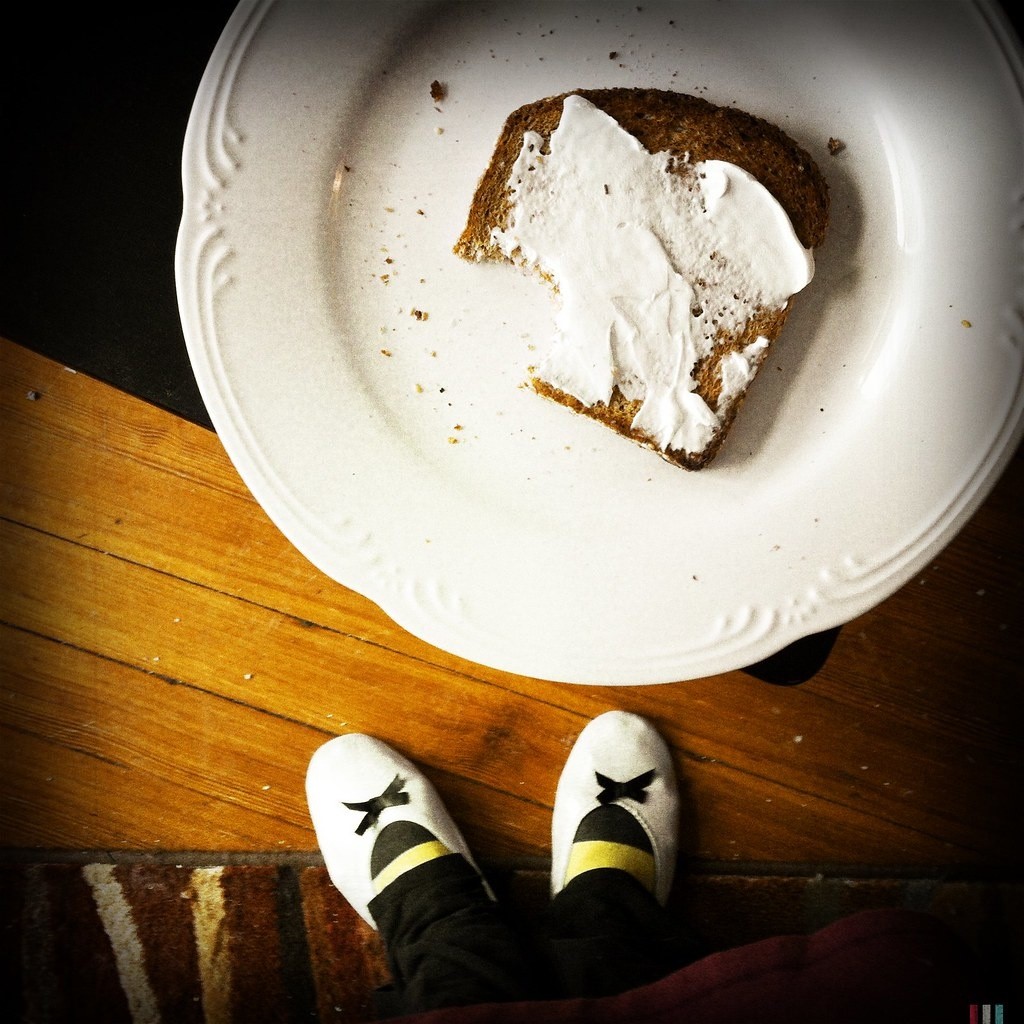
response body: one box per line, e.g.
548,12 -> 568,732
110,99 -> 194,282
174,0 -> 1024,686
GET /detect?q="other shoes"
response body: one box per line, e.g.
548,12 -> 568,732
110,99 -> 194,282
550,710 -> 682,907
305,733 -> 497,936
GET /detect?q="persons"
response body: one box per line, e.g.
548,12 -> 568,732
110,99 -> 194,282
303,708 -> 703,1022
